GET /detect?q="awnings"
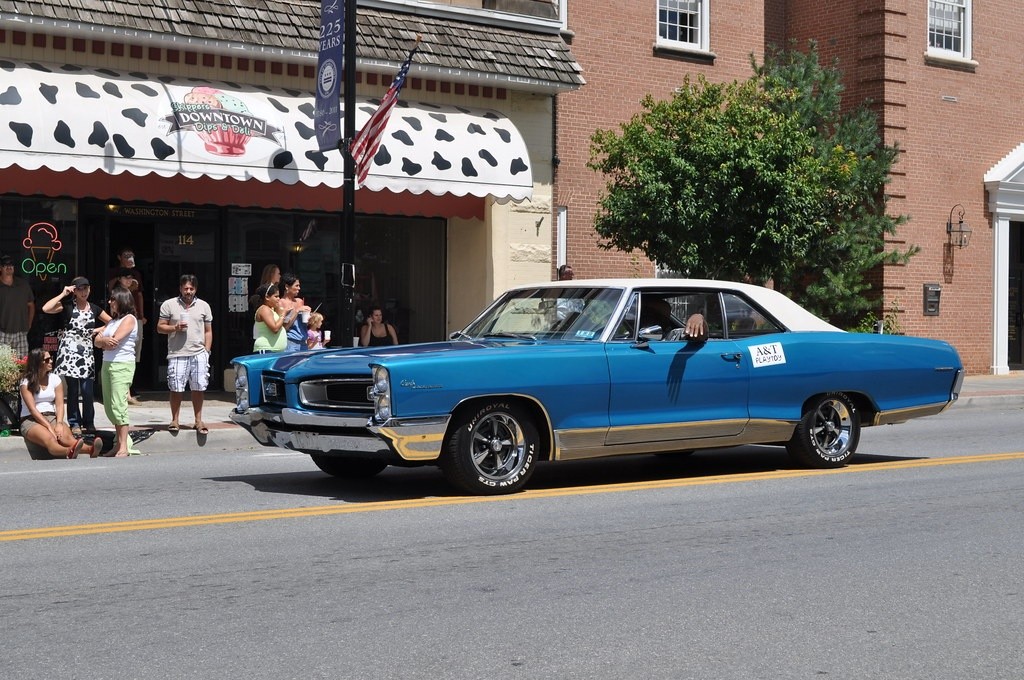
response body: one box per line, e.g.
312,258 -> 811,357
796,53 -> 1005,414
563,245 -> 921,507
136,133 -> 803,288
0,62 -> 534,208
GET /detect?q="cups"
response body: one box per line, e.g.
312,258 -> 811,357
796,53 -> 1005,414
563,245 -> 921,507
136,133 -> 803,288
180,313 -> 188,322
352,337 -> 359,347
302,311 -> 310,323
324,331 -> 331,340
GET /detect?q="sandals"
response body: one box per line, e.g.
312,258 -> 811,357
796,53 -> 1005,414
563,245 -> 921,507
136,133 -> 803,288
103,446 -> 119,457
115,451 -> 130,456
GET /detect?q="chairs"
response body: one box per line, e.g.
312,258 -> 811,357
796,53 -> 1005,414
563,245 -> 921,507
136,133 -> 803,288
736,318 -> 756,330
649,300 -> 672,331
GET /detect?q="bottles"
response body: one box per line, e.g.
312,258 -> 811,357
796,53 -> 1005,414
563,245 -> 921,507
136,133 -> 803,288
1,429 -> 11,437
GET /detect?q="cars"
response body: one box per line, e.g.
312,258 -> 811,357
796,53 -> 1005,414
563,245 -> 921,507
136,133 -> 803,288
228,279 -> 963,496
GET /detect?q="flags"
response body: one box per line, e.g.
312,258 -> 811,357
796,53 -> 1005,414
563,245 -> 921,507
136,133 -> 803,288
348,48 -> 417,187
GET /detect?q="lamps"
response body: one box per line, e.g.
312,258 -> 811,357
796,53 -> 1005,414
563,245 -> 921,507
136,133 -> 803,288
947,203 -> 972,253
291,230 -> 305,257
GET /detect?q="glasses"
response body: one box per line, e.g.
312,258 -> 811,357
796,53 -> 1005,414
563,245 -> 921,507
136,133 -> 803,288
42,356 -> 53,363
109,296 -> 116,301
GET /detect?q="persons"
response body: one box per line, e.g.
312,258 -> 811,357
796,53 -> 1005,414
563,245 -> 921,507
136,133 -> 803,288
117,267 -> 142,407
250,264 -> 330,354
556,265 -> 585,322
42,276 -> 112,436
157,274 -> 212,434
95,286 -> 139,457
0,255 -> 34,362
108,249 -> 146,363
641,300 -> 710,343
361,307 -> 398,346
19,349 -> 103,458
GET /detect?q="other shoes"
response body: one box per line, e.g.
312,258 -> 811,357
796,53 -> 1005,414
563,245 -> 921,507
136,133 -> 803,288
89,437 -> 103,458
71,427 -> 82,434
128,399 -> 142,406
81,426 -> 96,433
66,438 -> 84,459
132,392 -> 140,397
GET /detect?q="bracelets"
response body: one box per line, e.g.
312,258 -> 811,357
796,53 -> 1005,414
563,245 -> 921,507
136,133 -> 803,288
57,422 -> 63,425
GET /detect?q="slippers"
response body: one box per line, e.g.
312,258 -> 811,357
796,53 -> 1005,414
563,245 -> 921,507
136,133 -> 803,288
168,422 -> 179,431
194,422 -> 208,434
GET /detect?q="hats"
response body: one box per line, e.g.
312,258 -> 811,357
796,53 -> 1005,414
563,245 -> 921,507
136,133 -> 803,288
118,269 -> 135,278
0,255 -> 13,264
71,277 -> 89,286
